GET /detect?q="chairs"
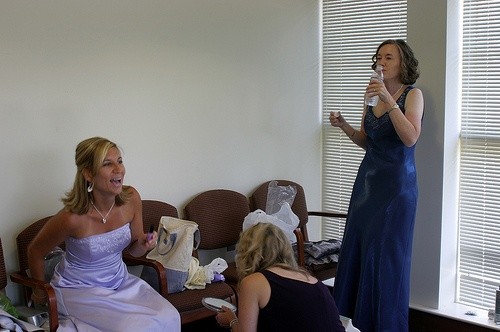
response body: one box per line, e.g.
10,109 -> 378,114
9,215 -> 167,332
183,189 -> 254,314
124,200 -> 236,324
251,180 -> 347,280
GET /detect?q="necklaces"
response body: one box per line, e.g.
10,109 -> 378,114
89,199 -> 115,224
392,83 -> 404,98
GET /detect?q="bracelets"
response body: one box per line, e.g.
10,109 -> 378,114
349,130 -> 355,138
32,290 -> 48,299
229,319 -> 238,328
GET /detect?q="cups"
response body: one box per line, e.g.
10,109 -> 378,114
488,309 -> 495,320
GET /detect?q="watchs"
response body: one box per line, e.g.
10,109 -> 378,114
388,104 -> 399,114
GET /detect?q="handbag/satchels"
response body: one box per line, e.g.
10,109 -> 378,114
140,216 -> 201,293
15,306 -> 48,327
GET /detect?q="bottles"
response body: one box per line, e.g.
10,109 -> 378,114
494,285 -> 500,323
365,65 -> 383,106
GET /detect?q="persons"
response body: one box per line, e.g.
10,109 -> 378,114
27,137 -> 181,332
215,222 -> 346,332
329,39 -> 426,332
